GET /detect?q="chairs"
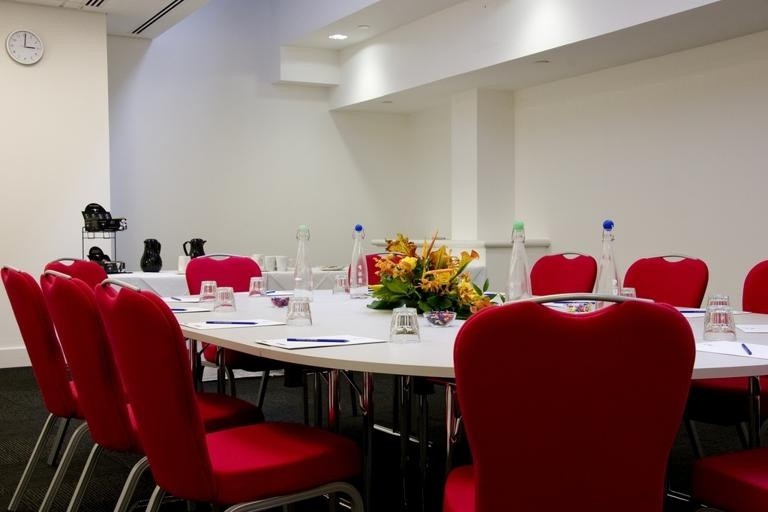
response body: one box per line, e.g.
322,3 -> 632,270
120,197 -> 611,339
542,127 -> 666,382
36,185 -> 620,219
346,251 -> 447,471
697,260 -> 768,444
530,252 -> 597,296
623,254 -> 709,309
185,254 -> 322,425
43,256 -> 108,284
40,270 -> 263,512
92,277 -> 367,512
694,446 -> 768,512
442,291 -> 695,512
0,266 -> 84,512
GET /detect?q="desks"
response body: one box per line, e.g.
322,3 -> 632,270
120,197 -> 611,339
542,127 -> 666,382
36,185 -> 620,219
160,287 -> 768,512
105,261 -> 359,382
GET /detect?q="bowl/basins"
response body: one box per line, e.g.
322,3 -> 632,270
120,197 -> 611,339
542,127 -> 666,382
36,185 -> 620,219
427,310 -> 453,325
85,217 -> 128,231
271,295 -> 295,307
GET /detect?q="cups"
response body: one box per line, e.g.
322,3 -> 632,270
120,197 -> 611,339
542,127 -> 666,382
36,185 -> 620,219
287,297 -> 312,326
265,255 -> 295,272
214,287 -> 237,314
621,287 -> 636,298
199,281 -> 218,303
333,275 -> 349,296
705,296 -> 738,346
387,308 -> 422,345
103,262 -> 126,272
178,256 -> 190,274
248,276 -> 265,298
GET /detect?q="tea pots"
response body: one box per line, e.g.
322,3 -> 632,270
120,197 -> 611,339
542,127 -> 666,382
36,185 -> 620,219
183,238 -> 206,259
252,254 -> 265,271
140,238 -> 162,272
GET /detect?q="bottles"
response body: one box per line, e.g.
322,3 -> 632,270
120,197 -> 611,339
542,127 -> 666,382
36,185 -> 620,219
293,224 -> 312,302
508,220 -> 533,303
348,223 -> 368,297
592,219 -> 621,310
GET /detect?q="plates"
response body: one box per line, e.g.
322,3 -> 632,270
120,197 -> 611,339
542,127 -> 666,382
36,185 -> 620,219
320,265 -> 346,270
81,203 -> 111,231
89,247 -> 104,263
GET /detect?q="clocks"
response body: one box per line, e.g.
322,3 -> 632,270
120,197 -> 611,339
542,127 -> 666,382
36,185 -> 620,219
6,29 -> 45,66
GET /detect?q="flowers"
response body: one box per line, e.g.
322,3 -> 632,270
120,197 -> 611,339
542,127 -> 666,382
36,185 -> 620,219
369,234 -> 502,319
